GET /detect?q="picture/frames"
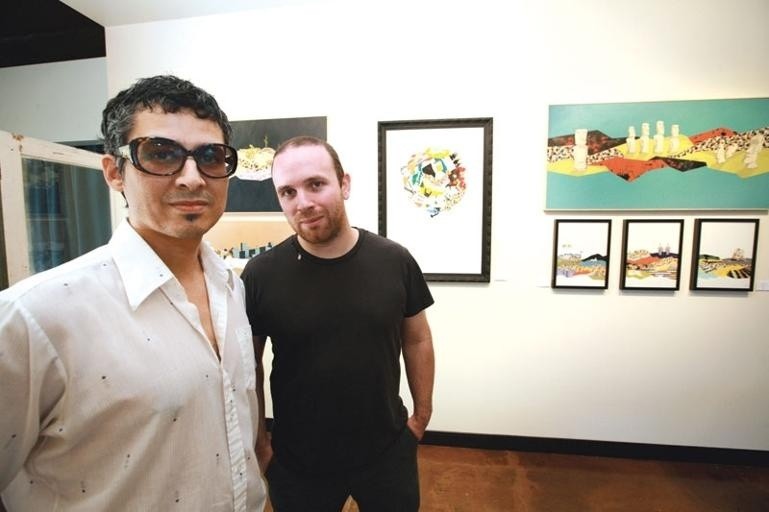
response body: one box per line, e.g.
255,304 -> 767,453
550,218 -> 761,293
377,116 -> 493,283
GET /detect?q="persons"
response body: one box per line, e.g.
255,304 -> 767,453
240,135 -> 435,510
2,71 -> 269,512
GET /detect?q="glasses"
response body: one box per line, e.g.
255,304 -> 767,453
114,135 -> 238,180
